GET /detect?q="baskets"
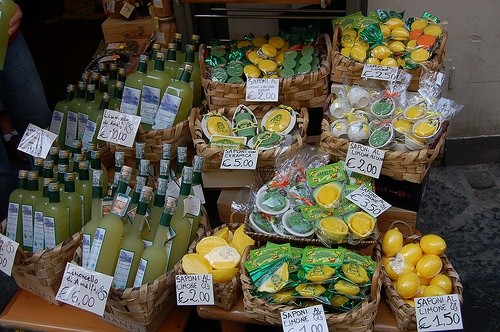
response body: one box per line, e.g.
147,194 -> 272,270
0,24 -> 463,332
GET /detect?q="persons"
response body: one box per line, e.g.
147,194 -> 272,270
0,0 -> 53,172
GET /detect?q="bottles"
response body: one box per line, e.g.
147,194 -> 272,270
82,141 -> 204,289
119,32 -> 202,130
50,41 -> 127,152
5,139 -> 108,253
0,0 -> 16,70
